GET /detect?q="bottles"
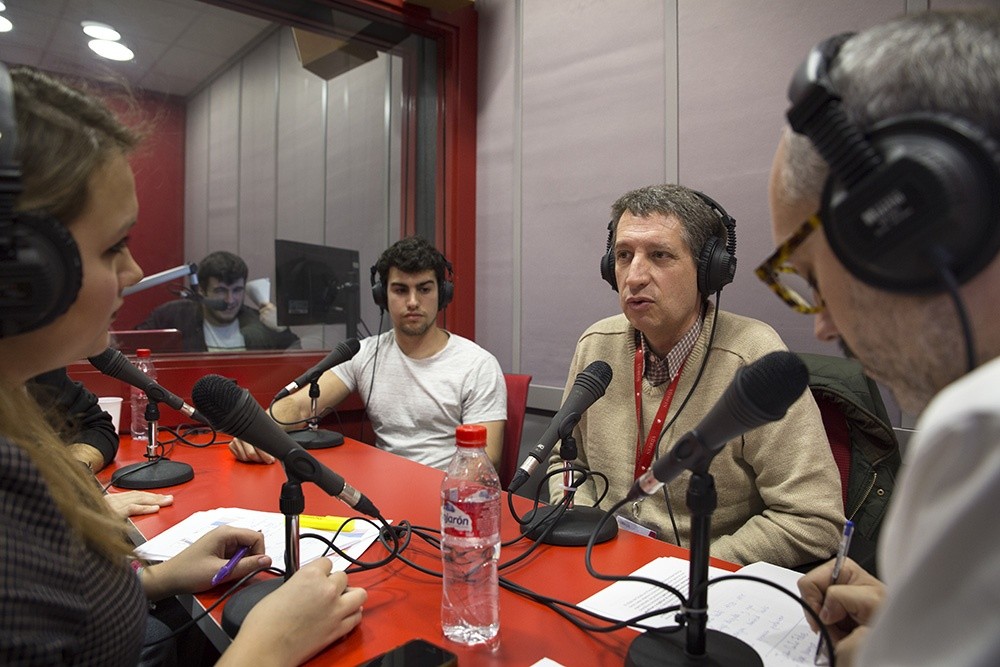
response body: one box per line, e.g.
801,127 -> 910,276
130,348 -> 158,441
439,424 -> 501,646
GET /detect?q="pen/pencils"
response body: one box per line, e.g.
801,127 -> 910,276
813,520 -> 855,665
212,529 -> 263,588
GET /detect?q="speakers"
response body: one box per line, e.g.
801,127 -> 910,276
289,25 -> 380,82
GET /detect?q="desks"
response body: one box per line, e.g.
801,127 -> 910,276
91,425 -> 846,667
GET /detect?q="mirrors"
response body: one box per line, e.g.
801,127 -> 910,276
1,3 -> 406,352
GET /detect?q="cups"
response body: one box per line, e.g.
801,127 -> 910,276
97,397 -> 123,434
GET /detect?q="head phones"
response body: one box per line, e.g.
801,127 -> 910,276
786,23 -> 1000,295
599,186 -> 737,304
0,64 -> 85,346
370,235 -> 455,314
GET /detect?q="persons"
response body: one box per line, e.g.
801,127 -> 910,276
19,367 -> 121,475
130,251 -> 308,354
764,26 -> 1000,667
228,238 -> 508,485
0,62 -> 372,666
537,178 -> 852,570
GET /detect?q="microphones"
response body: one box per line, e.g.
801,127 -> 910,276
168,287 -> 229,312
626,348 -> 809,503
87,347 -> 213,429
508,360 -> 612,493
273,335 -> 362,404
192,373 -> 381,519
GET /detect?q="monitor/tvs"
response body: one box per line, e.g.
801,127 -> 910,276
274,238 -> 363,326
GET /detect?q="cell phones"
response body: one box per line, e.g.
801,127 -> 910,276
356,636 -> 458,667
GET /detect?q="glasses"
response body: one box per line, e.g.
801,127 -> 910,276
755,214 -> 825,315
